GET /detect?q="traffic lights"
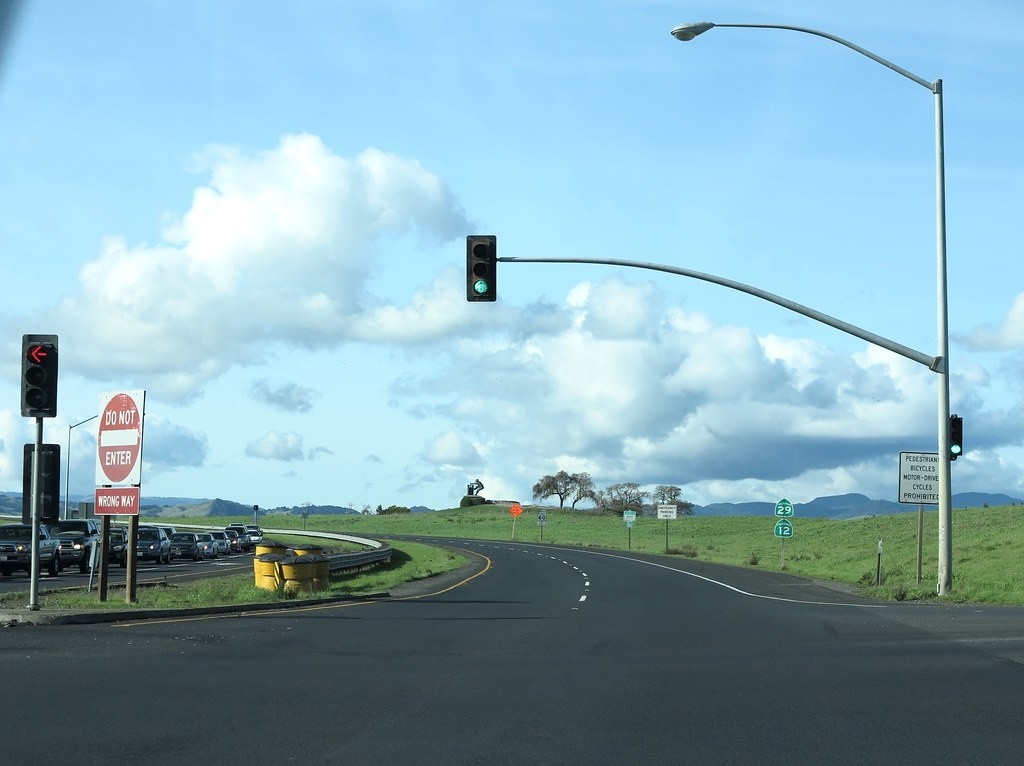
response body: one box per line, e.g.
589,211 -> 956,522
466,234 -> 497,304
22,335 -> 58,418
947,414 -> 963,457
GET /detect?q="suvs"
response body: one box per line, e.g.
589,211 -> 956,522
109,524 -> 176,568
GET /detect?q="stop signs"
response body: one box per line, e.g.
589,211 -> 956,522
95,390 -> 145,484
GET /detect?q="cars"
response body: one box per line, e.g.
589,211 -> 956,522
225,523 -> 262,554
170,531 -> 232,561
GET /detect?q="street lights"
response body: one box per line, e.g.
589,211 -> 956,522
671,21 -> 952,598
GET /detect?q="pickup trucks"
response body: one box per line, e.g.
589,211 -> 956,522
0,520 -> 101,577
